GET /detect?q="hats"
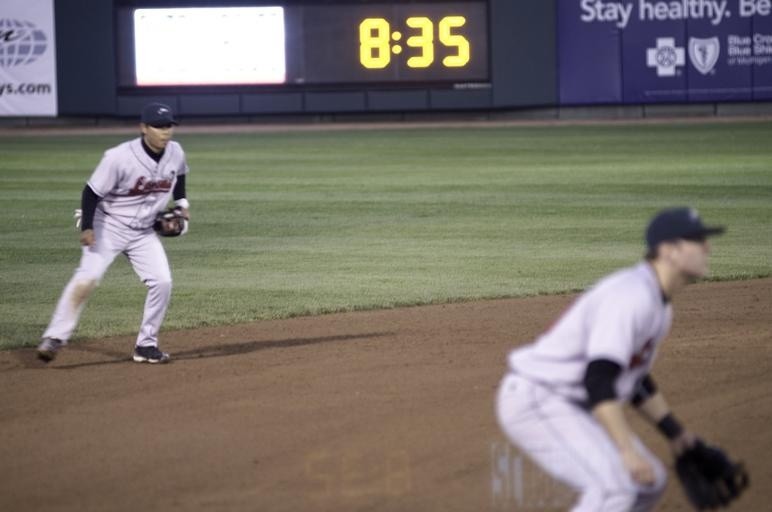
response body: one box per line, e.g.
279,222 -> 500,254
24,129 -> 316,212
648,208 -> 727,241
141,103 -> 179,126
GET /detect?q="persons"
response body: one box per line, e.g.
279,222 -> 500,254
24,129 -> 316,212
496,205 -> 750,512
35,102 -> 190,365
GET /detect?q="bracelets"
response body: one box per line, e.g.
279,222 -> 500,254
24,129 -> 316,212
654,411 -> 684,441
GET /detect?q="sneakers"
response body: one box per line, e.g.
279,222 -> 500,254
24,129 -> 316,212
36,338 -> 62,362
133,345 -> 169,365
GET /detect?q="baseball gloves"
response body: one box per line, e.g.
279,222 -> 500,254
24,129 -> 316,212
675,438 -> 749,510
153,206 -> 183,236
